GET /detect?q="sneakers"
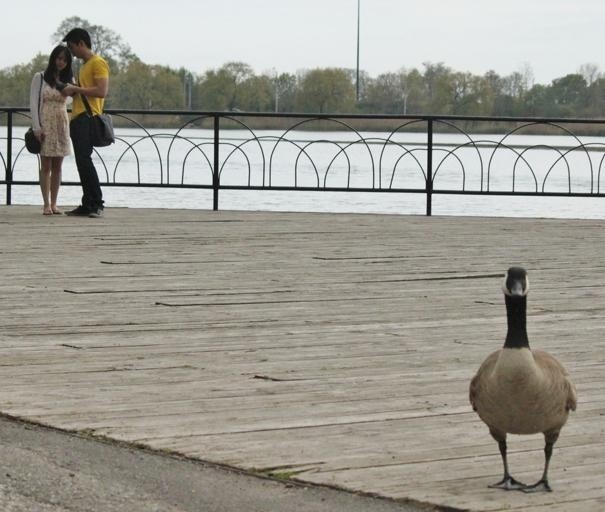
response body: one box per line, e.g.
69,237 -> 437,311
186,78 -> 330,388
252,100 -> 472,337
65,204 -> 104,216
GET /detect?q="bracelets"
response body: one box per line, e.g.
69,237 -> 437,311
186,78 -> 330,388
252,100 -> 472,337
70,85 -> 74,94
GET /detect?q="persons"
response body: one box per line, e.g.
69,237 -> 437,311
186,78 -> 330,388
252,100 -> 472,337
28,46 -> 77,215
61,28 -> 112,218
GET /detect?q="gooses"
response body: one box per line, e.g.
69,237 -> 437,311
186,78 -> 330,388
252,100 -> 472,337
468,267 -> 577,493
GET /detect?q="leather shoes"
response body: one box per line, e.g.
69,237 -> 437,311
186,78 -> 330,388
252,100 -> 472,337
43,208 -> 61,214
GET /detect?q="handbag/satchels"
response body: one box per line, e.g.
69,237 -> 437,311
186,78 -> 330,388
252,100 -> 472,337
90,113 -> 115,147
25,128 -> 41,154
